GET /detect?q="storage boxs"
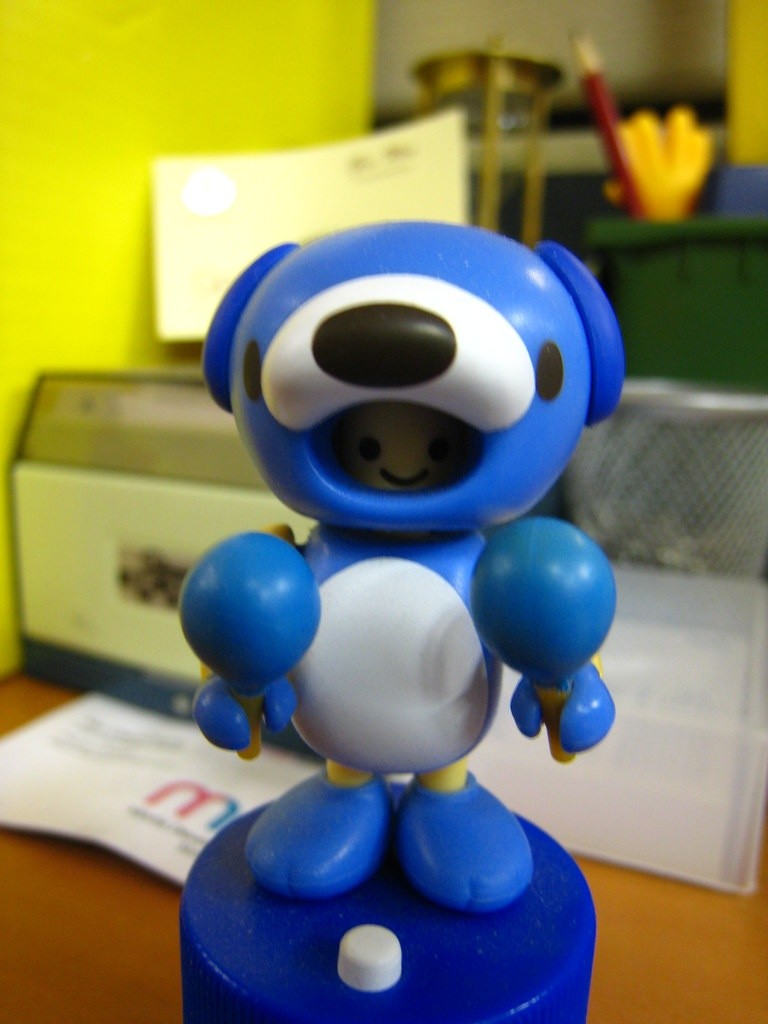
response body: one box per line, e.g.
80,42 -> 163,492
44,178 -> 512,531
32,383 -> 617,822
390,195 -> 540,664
12,462 -> 317,741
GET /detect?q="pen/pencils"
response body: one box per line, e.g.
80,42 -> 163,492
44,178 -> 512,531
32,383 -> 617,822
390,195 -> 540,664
561,30 -> 647,219
